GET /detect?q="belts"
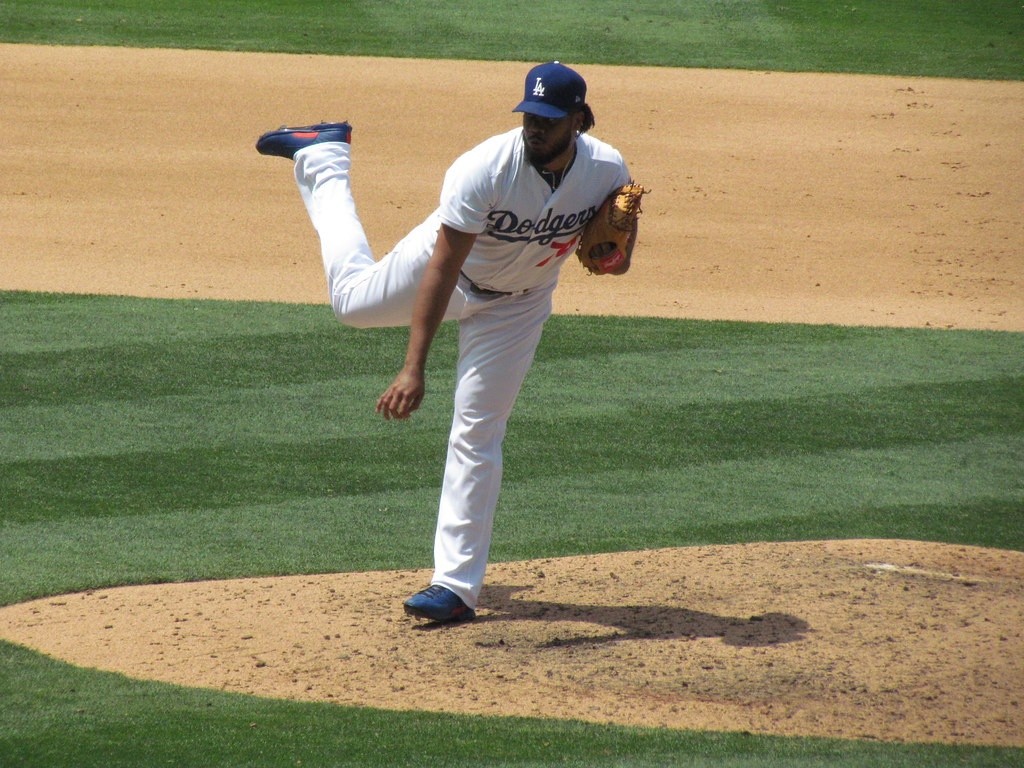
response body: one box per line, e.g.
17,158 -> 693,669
458,269 -> 528,295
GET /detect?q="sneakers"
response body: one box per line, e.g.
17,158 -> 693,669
256,120 -> 352,160
404,585 -> 476,621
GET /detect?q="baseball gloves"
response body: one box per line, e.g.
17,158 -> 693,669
573,178 -> 654,278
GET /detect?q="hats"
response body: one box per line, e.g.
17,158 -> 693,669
512,61 -> 587,117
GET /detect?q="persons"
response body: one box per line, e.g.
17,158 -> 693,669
256,61 -> 643,622
